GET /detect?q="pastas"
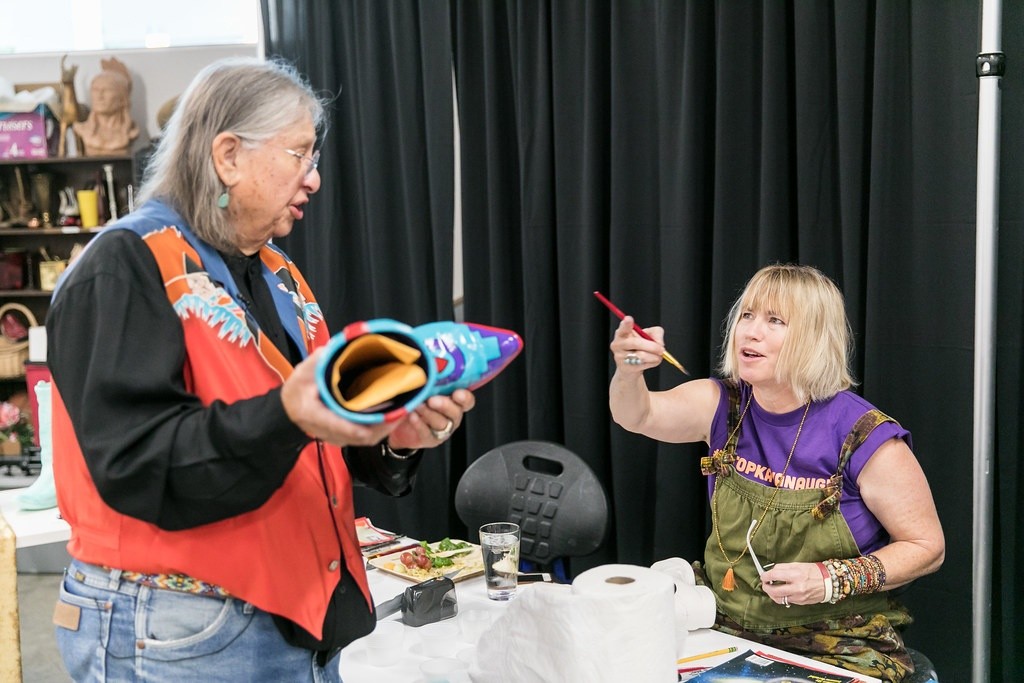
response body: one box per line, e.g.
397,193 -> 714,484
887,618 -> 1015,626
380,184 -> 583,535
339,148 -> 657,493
399,563 -> 444,581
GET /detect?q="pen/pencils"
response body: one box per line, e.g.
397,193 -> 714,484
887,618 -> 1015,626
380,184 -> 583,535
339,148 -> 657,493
678,666 -> 710,674
676,646 -> 739,664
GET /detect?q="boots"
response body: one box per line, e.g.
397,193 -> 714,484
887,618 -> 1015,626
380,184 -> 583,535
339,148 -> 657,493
315,318 -> 523,425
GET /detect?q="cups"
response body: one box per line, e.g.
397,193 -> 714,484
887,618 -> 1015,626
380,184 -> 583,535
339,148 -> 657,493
39,261 -> 67,291
77,189 -> 98,227
478,522 -> 521,601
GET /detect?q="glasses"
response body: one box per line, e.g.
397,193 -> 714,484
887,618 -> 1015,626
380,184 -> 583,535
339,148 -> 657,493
241,136 -> 320,178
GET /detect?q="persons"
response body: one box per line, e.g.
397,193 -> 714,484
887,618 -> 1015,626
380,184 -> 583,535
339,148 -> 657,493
73,71 -> 140,154
46,61 -> 474,682
609,265 -> 945,683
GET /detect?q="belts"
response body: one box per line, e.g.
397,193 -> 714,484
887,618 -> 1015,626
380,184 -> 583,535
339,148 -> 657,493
103,566 -> 231,598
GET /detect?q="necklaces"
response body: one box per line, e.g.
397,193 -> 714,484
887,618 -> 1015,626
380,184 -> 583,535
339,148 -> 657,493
713,390 -> 811,591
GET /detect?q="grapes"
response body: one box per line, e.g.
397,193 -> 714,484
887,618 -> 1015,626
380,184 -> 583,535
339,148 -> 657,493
399,546 -> 433,571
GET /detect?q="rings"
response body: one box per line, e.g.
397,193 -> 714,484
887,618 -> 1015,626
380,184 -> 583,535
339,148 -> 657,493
782,596 -> 792,608
624,353 -> 641,365
431,418 -> 454,439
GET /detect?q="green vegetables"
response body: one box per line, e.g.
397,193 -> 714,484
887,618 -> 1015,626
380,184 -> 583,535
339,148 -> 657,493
419,538 -> 473,567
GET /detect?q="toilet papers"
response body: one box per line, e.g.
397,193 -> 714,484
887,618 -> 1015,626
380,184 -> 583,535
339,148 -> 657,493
473,561 -> 680,683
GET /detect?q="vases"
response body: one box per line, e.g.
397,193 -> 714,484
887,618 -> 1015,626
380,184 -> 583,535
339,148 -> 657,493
0,432 -> 23,456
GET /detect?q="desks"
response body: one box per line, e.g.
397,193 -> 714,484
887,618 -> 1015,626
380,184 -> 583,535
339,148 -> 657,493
335,574 -> 884,683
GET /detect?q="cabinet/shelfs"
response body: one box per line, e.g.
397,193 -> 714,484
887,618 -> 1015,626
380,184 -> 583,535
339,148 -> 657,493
0,137 -> 155,461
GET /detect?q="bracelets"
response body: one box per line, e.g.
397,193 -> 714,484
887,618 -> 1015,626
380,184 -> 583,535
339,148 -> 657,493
387,446 -> 418,459
817,555 -> 886,604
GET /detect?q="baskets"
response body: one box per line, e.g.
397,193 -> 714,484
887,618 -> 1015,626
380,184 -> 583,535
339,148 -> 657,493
0,303 -> 36,379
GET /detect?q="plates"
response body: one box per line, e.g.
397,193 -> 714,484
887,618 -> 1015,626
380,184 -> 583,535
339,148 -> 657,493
366,539 -> 484,582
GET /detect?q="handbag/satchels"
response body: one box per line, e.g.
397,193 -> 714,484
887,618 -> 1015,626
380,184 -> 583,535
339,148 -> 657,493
466,564 -> 683,683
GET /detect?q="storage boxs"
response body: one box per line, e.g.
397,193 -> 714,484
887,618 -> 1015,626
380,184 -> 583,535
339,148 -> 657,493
0,82 -> 62,160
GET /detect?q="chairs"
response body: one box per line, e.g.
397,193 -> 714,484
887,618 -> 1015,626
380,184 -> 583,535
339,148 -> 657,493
455,440 -> 607,583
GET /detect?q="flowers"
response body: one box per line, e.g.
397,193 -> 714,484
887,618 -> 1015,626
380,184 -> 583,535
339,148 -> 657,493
0,402 -> 34,451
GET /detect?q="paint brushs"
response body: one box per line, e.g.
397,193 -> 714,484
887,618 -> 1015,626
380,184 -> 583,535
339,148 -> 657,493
594,288 -> 689,375
